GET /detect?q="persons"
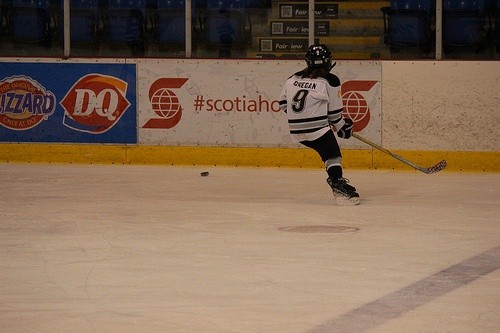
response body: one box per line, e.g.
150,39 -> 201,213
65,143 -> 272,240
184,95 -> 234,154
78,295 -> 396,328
279,43 -> 360,198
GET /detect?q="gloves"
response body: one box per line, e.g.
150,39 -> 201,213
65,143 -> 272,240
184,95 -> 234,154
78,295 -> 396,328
338,118 -> 353,139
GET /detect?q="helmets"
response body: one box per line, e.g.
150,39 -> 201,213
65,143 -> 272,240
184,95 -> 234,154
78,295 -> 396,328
305,45 -> 337,72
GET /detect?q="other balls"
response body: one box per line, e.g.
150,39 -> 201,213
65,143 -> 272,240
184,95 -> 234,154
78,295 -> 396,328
201,171 -> 209,177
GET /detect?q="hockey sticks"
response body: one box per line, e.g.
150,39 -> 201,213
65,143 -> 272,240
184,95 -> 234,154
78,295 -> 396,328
350,130 -> 448,175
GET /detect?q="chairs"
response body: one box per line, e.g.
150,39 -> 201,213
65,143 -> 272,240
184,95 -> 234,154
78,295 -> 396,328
441,8 -> 491,55
101,8 -> 149,50
198,8 -> 253,50
150,7 -> 198,51
380,6 -> 430,55
4,4 -> 57,48
59,8 -> 96,46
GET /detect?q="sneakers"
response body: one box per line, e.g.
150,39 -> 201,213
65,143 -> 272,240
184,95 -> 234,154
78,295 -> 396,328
327,177 -> 360,205
327,178 -> 357,191
